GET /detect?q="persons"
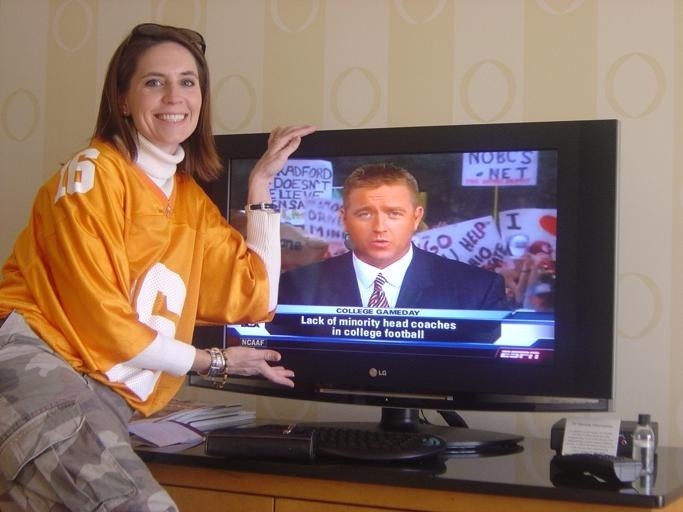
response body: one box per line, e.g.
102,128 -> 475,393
0,21 -> 319,511
265,162 -> 504,345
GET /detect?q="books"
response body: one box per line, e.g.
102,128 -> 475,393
125,399 -> 258,451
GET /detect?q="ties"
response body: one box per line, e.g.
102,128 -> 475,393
367,272 -> 390,307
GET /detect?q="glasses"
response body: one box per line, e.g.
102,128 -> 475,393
131,23 -> 206,56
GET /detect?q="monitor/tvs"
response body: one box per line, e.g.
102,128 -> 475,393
187,119 -> 619,450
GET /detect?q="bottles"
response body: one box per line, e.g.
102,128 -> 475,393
628,413 -> 657,479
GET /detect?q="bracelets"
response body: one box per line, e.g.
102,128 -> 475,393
241,203 -> 277,213
197,347 -> 227,390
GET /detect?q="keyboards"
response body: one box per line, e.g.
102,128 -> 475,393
204,429 -> 446,460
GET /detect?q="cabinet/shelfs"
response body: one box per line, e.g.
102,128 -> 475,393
126,416 -> 683,512
